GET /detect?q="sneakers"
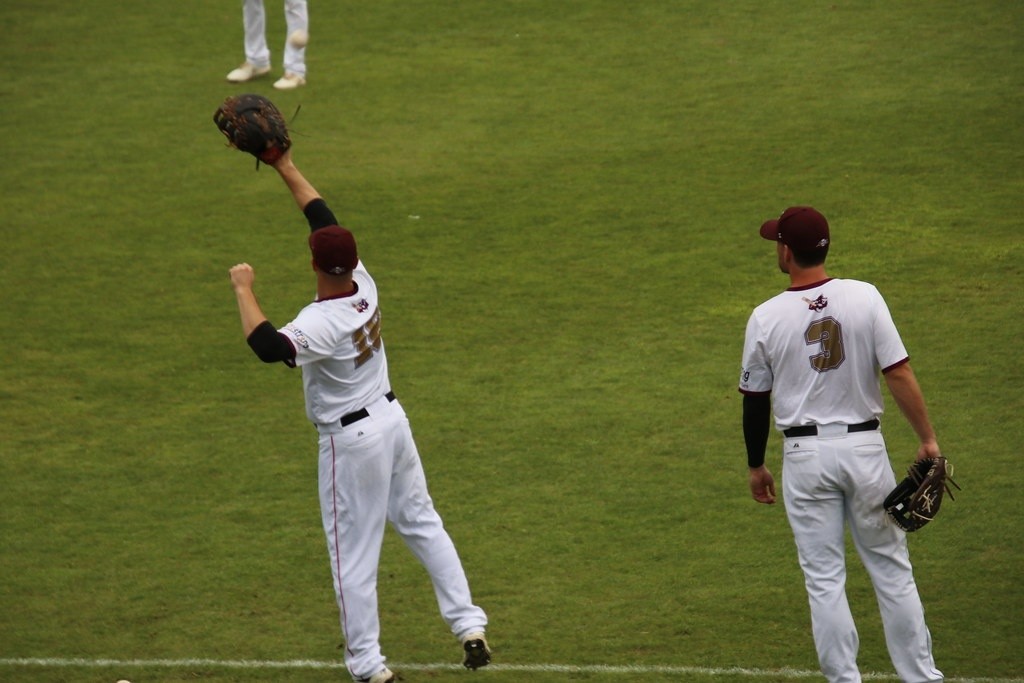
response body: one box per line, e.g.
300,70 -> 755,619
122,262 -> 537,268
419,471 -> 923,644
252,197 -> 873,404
354,665 -> 395,683
462,634 -> 491,671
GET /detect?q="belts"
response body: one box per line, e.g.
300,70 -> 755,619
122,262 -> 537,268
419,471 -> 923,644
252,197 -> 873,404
783,419 -> 879,437
313,389 -> 396,428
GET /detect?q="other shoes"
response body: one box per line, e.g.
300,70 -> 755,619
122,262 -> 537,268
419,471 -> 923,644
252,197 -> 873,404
226,60 -> 272,82
273,71 -> 306,89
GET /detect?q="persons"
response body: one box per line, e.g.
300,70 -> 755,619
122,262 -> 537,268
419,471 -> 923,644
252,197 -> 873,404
738,207 -> 948,683
227,0 -> 309,89
212,94 -> 490,683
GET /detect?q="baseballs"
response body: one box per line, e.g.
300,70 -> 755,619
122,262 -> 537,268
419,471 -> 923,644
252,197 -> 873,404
289,30 -> 309,48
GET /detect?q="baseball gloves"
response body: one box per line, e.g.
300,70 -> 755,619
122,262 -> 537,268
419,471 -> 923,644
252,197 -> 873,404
211,93 -> 303,173
881,454 -> 963,534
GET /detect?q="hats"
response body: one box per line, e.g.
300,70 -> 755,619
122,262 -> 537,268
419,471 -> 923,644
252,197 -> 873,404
308,225 -> 359,276
759,206 -> 830,251
